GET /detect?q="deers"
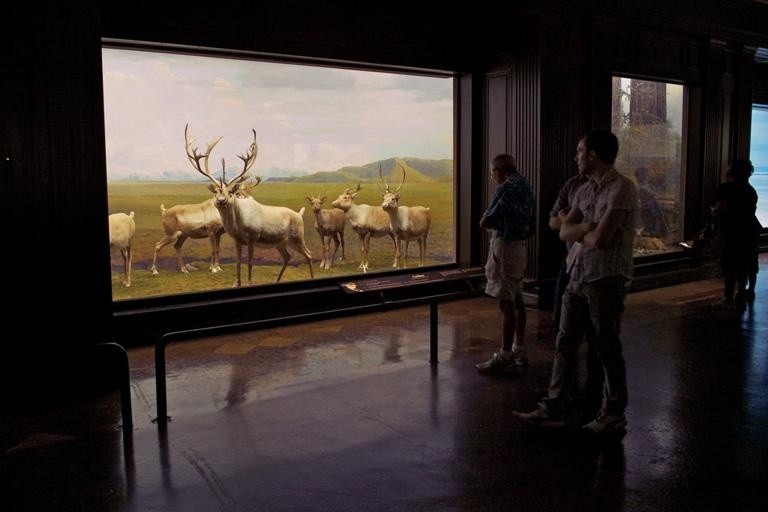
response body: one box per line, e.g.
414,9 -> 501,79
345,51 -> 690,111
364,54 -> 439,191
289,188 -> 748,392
375,161 -> 432,270
331,179 -> 397,275
108,210 -> 137,288
149,175 -> 263,275
183,123 -> 314,288
303,191 -> 347,271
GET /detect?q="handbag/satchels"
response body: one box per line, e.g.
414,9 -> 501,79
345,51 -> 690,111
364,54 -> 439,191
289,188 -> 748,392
692,202 -> 719,266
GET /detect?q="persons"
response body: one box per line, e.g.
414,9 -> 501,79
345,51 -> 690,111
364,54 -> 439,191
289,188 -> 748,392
474,155 -> 533,370
703,157 -> 762,302
512,130 -> 638,433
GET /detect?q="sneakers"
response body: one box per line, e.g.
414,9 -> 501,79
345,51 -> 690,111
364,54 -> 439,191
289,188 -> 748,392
476,353 -> 528,375
583,413 -> 628,434
513,407 -> 564,427
